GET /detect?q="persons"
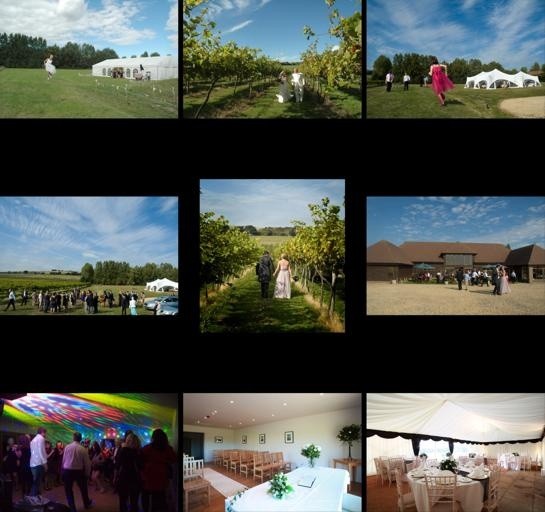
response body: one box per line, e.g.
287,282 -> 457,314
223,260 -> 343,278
428,57 -> 456,108
258,250 -> 275,298
411,263 -> 510,297
20,287 -> 146,315
272,253 -> 294,299
290,68 -> 305,103
402,73 -> 411,91
383,70 -> 394,92
43,54 -> 56,82
3,287 -> 16,312
1,427 -> 178,509
277,71 -> 291,104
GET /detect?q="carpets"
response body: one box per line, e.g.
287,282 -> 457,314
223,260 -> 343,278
196,467 -> 251,499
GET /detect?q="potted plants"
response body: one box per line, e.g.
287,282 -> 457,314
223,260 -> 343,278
336,423 -> 361,460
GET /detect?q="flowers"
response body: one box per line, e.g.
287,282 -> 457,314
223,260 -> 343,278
300,441 -> 323,459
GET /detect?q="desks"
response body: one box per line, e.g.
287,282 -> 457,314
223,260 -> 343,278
331,457 -> 362,491
223,460 -> 350,512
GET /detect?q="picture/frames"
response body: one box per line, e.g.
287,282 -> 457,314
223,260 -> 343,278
214,435 -> 223,443
241,434 -> 247,444
284,430 -> 294,443
258,433 -> 266,444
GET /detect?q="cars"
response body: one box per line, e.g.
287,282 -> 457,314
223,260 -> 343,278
156,301 -> 178,315
144,295 -> 178,311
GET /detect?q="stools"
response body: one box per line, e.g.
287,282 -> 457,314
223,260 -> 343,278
343,492 -> 362,512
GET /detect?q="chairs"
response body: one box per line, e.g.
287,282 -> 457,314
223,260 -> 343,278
373,449 -> 538,512
182,447 -> 292,512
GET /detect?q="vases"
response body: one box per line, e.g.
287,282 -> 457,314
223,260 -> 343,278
308,455 -> 315,469
